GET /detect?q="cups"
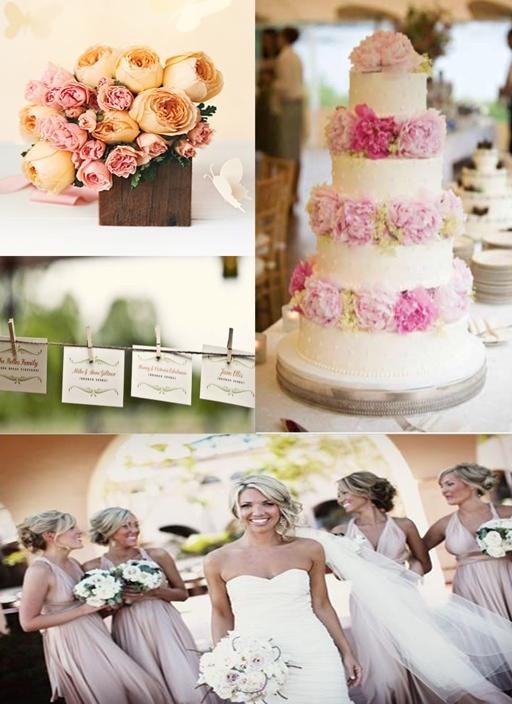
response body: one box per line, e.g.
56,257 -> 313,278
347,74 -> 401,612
254,332 -> 267,365
452,229 -> 511,305
282,302 -> 300,329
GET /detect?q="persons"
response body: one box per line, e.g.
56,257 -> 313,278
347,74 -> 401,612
498,30 -> 512,154
256,27 -> 304,217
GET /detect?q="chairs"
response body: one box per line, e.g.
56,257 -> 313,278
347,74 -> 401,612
255,153 -> 297,328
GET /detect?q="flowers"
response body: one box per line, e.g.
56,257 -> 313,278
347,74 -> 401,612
349,29 -> 433,76
15,40 -> 225,195
114,558 -> 171,590
285,256 -> 477,334
304,181 -> 470,257
324,101 -> 450,159
72,567 -> 128,609
473,517 -> 512,559
185,628 -> 307,703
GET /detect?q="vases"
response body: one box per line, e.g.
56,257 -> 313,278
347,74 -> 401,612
96,158 -> 193,227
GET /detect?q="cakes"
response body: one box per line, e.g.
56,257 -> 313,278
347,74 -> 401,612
287,30 -> 474,383
